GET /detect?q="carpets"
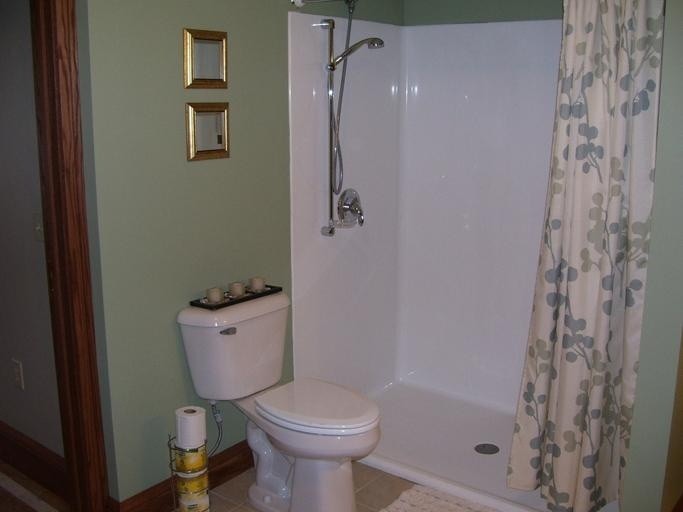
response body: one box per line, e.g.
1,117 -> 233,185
378,482 -> 496,512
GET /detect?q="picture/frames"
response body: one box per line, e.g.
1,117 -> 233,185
187,100 -> 231,163
182,26 -> 229,90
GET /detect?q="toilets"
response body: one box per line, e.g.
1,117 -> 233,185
176,290 -> 381,512
